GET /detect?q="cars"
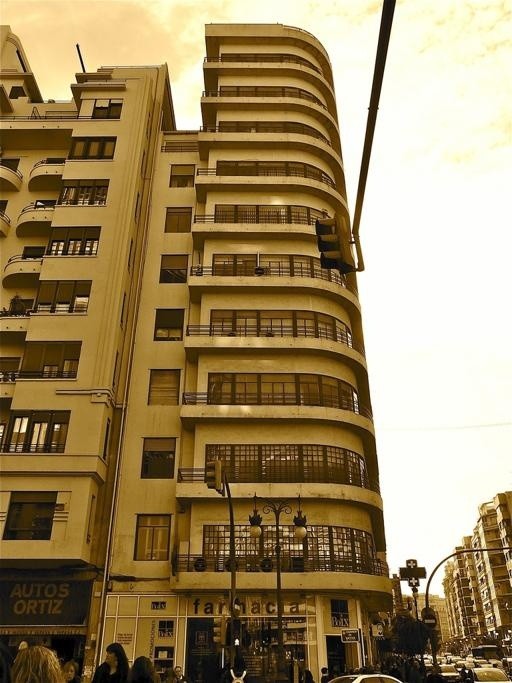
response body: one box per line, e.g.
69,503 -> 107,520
416,652 -> 512,682
328,674 -> 404,683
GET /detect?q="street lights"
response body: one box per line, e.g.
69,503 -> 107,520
248,491 -> 308,683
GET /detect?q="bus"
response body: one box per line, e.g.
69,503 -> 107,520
472,645 -> 503,660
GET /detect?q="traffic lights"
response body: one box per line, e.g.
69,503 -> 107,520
205,460 -> 222,492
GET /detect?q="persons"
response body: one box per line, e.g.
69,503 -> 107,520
224,654 -> 252,683
171,666 -> 193,683
8,644 -> 67,682
320,667 -> 330,682
128,656 -> 162,682
302,670 -> 315,682
64,658 -> 83,682
91,642 -> 129,682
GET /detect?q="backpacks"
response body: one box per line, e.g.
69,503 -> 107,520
230,668 -> 247,683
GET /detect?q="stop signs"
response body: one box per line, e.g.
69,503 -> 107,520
421,614 -> 437,629
